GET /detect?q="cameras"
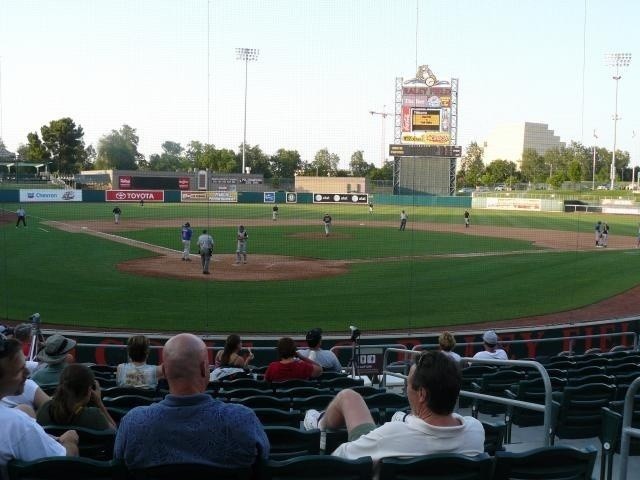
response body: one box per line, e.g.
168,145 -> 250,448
28,312 -> 42,330
348,323 -> 361,340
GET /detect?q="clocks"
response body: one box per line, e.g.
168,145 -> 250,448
425,77 -> 436,87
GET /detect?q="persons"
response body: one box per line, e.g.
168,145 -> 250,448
115,335 -> 163,390
464,208 -> 471,227
6,379 -> 51,419
272,205 -> 278,220
298,328 -> 343,372
13,323 -> 32,361
0,339 -> 80,466
322,213 -> 332,237
31,334 -> 77,397
214,334 -> 254,368
236,225 -> 249,264
601,223 -> 609,247
15,205 -> 27,229
369,201 -> 373,215
473,330 -> 509,360
264,337 -> 323,382
113,332 -> 270,466
180,221 -> 194,262
595,220 -> 602,247
197,229 -> 214,275
399,210 -> 408,231
303,351 -> 486,479
36,363 -> 117,431
438,331 -> 461,361
112,206 -> 122,224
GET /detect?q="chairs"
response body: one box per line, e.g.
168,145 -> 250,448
618,383 -> 640,401
317,369 -> 347,377
550,384 -> 617,445
456,366 -> 498,410
502,377 -> 567,444
325,426 -> 358,453
568,374 -> 616,384
494,446 -> 597,480
577,359 -> 609,366
370,408 -> 380,423
101,395 -> 163,410
481,419 -> 506,453
254,454 -> 375,480
617,371 -> 640,382
361,391 -> 409,407
221,395 -> 291,410
122,462 -> 253,480
526,369 -> 567,378
383,405 -> 411,423
43,426 -> 116,454
253,365 -> 270,374
579,354 -> 601,359
320,377 -> 364,388
91,370 -> 116,380
544,361 -> 577,368
38,382 -> 60,398
377,456 -> 498,480
465,371 -> 525,420
270,379 -> 317,389
2,456 -> 113,480
606,352 -> 628,357
221,372 -> 253,379
219,389 -> 274,398
276,385 -> 331,397
91,376 -> 118,388
335,386 -> 385,395
100,385 -> 158,397
568,365 -> 607,375
599,406 -> 639,480
251,405 -> 301,428
261,426 -> 321,453
291,393 -> 338,410
88,365 -> 116,372
608,363 -> 640,371
609,354 -> 640,365
105,406 -> 128,425
207,381 -> 222,389
222,377 -> 274,388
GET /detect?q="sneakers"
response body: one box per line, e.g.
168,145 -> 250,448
203,271 -> 211,274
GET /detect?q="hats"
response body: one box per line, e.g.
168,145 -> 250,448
303,408 -> 326,430
306,327 -> 324,343
483,330 -> 498,345
36,334 -> 76,363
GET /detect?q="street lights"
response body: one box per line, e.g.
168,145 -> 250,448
233,46 -> 259,174
602,51 -> 632,193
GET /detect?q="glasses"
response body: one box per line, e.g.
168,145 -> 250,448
418,350 -> 430,369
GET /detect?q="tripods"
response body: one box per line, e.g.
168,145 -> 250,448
345,340 -> 362,379
28,330 -> 46,363
